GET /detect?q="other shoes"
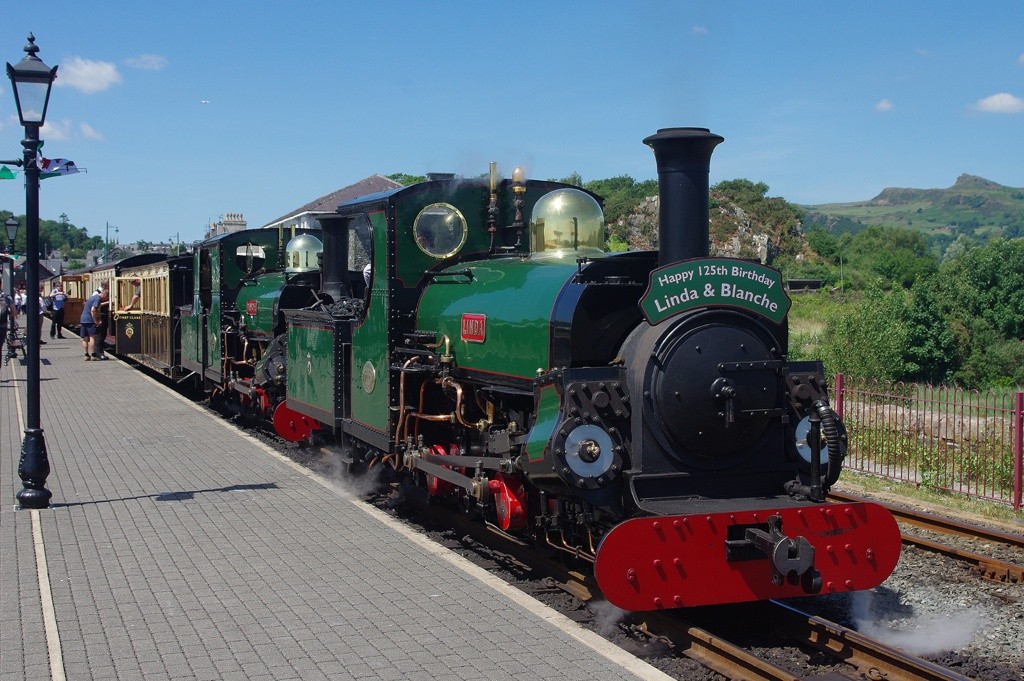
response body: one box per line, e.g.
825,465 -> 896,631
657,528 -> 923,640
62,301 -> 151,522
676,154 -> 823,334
97,353 -> 109,360
50,334 -> 55,339
58,335 -> 66,339
91,356 -> 101,361
84,356 -> 91,361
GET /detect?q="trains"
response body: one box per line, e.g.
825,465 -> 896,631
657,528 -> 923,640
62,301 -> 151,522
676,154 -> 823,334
37,124 -> 905,615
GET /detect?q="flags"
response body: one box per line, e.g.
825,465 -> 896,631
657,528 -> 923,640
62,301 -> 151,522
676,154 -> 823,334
36,139 -> 79,180
0,165 -> 19,179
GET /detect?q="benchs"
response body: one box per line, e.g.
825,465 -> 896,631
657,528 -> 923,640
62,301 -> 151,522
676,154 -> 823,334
3,319 -> 26,366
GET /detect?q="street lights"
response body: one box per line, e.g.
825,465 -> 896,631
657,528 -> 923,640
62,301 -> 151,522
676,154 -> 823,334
3,214 -> 21,359
104,220 -> 120,263
0,30 -> 60,511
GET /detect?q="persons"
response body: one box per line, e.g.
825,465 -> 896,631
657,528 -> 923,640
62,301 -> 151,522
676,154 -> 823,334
363,226 -> 436,289
0,290 -> 17,335
14,289 -> 27,316
124,274 -> 140,310
80,290 -> 109,360
50,282 -> 67,339
92,279 -> 109,360
39,292 -> 47,344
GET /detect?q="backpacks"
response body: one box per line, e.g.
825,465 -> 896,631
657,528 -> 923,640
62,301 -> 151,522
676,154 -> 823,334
46,289 -> 56,311
